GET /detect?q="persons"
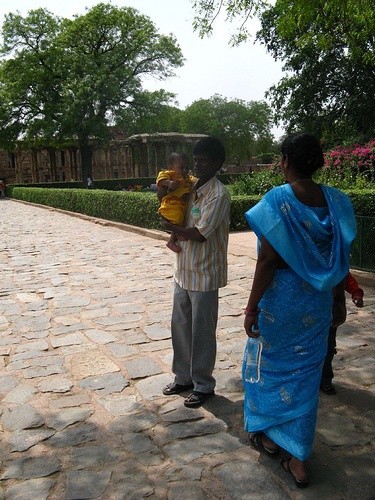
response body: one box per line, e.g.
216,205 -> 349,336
86,174 -> 94,189
0,177 -> 6,198
162,137 -> 231,408
114,183 -> 126,191
242,131 -> 357,488
128,181 -> 137,191
156,151 -> 199,253
319,272 -> 364,396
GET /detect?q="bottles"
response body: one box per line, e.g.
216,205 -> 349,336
192,207 -> 200,224
245,330 -> 263,383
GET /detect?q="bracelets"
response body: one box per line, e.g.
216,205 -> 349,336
244,306 -> 261,316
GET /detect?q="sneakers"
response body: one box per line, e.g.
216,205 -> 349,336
320,383 -> 337,395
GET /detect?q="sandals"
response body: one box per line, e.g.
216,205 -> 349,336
162,383 -> 194,395
184,390 -> 214,406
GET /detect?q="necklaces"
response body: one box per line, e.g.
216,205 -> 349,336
290,179 -> 314,186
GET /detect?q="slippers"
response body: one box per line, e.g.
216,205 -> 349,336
279,455 -> 309,488
249,432 -> 278,455
